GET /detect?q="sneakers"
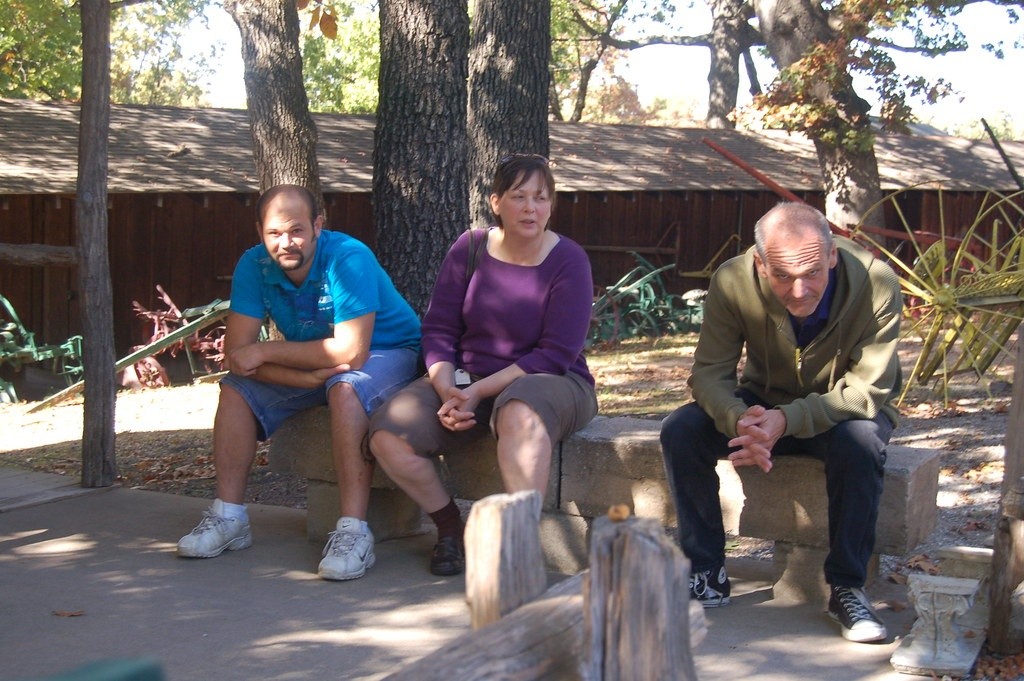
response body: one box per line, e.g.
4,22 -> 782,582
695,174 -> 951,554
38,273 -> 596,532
829,582 -> 886,643
177,498 -> 252,557
318,517 -> 375,580
689,567 -> 731,607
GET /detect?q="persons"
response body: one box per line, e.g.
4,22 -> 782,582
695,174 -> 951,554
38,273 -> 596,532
659,204 -> 903,641
177,185 -> 423,581
369,154 -> 599,577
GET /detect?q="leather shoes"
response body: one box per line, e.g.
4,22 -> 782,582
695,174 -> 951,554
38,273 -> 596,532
431,521 -> 466,575
